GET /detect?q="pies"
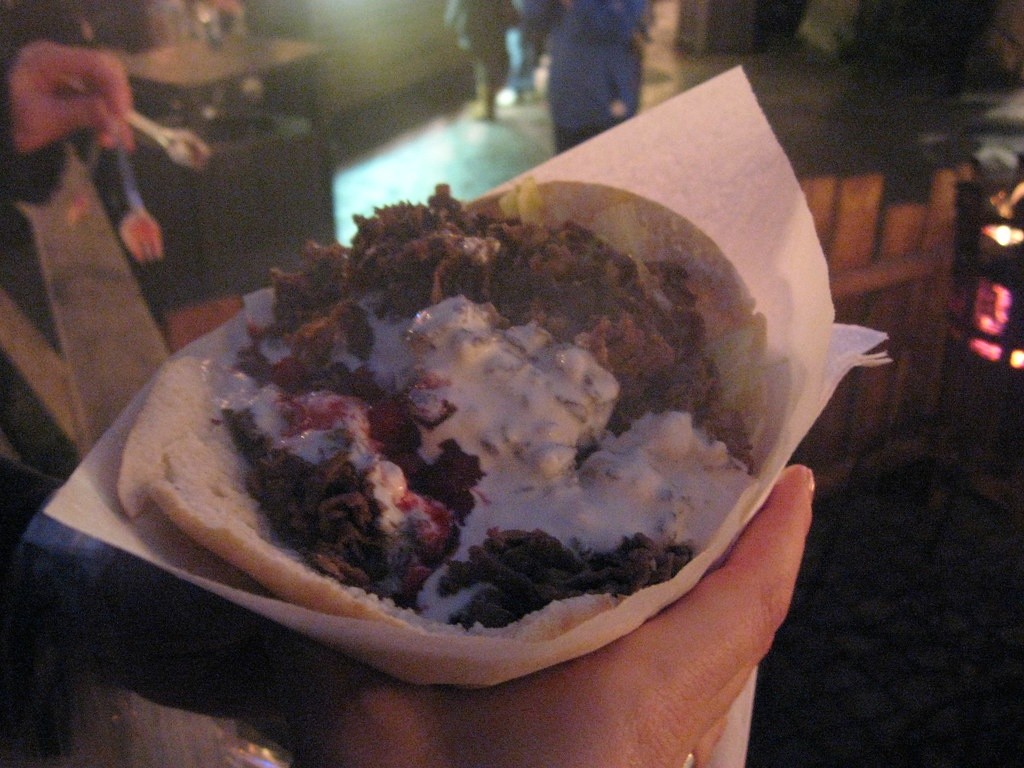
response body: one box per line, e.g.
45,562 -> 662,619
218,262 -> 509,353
108,176 -> 774,646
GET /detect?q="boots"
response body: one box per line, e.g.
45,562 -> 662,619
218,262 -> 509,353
467,81 -> 497,122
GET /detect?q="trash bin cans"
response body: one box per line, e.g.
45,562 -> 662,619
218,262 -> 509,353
191,114 -> 336,298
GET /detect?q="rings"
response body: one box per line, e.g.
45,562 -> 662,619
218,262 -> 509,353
683,753 -> 696,768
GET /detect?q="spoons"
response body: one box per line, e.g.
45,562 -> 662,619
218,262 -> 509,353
67,74 -> 209,168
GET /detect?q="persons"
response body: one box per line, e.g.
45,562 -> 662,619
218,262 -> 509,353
1,43 -> 814,768
445,0 -> 659,156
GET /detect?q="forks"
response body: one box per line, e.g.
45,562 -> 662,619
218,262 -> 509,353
96,98 -> 164,263
